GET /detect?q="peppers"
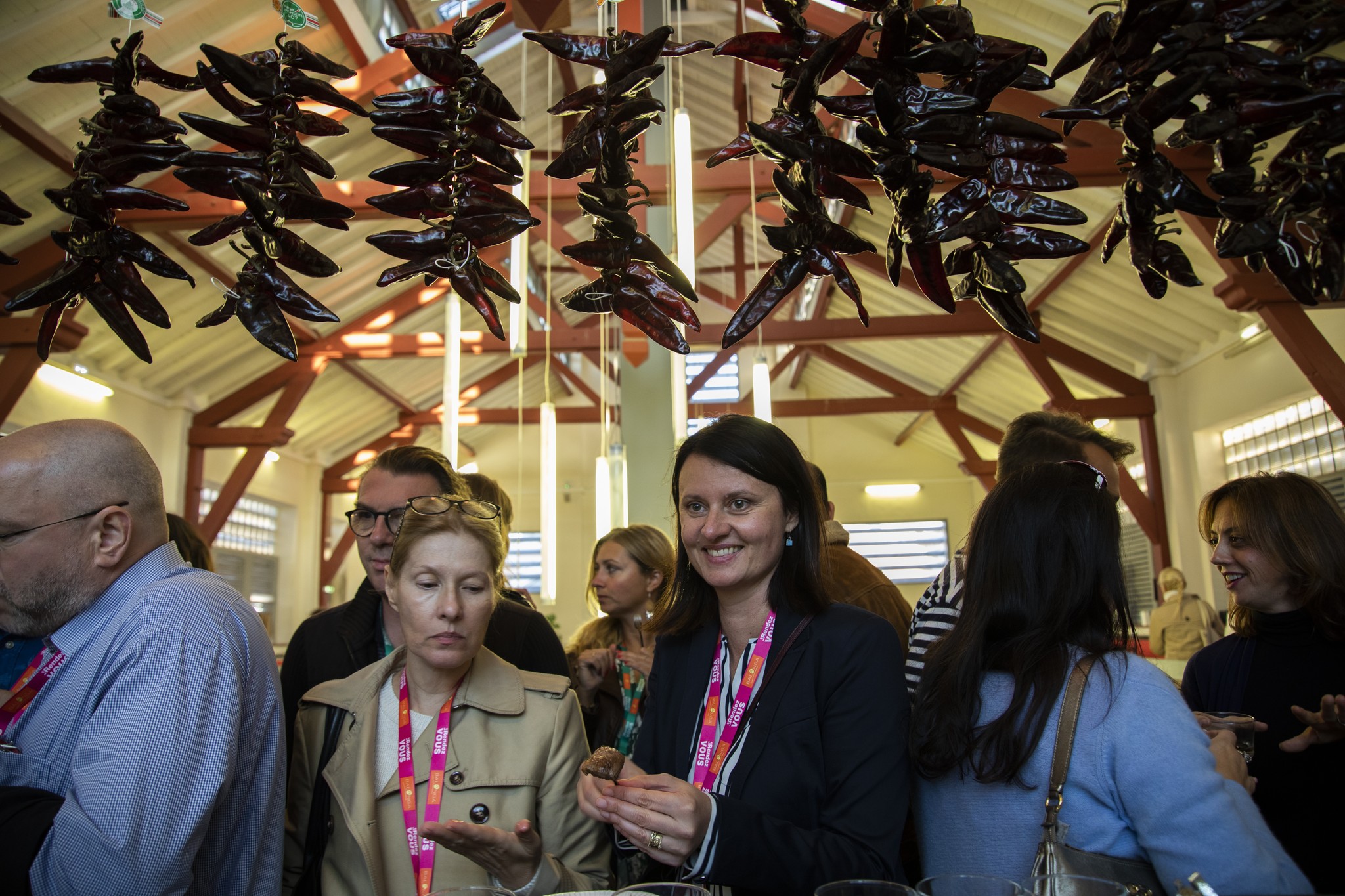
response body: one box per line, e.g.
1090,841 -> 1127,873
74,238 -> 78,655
0,0 -> 1342,367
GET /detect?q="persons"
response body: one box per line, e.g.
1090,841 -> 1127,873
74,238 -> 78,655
1147,565 -> 1225,661
564,522 -> 682,768
1,417 -> 287,896
805,461 -> 915,660
269,493 -> 613,896
571,411 -> 911,896
904,461 -> 1316,896
282,445 -> 571,741
1182,467 -> 1345,896
905,410 -> 1133,719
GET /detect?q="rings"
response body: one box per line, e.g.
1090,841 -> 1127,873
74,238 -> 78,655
648,830 -> 663,850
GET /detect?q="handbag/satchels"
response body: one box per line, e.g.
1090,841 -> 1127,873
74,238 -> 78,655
1030,842 -> 1166,896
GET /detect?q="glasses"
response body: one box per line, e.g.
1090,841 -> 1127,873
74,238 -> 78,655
345,507 -> 405,537
389,496 -> 502,565
1054,460 -> 1108,495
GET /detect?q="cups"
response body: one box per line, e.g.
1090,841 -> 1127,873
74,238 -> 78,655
408,884 -> 713,896
809,871 -> 1133,896
1199,709 -> 1258,763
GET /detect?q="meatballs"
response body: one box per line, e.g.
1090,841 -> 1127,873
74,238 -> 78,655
581,745 -> 625,781
